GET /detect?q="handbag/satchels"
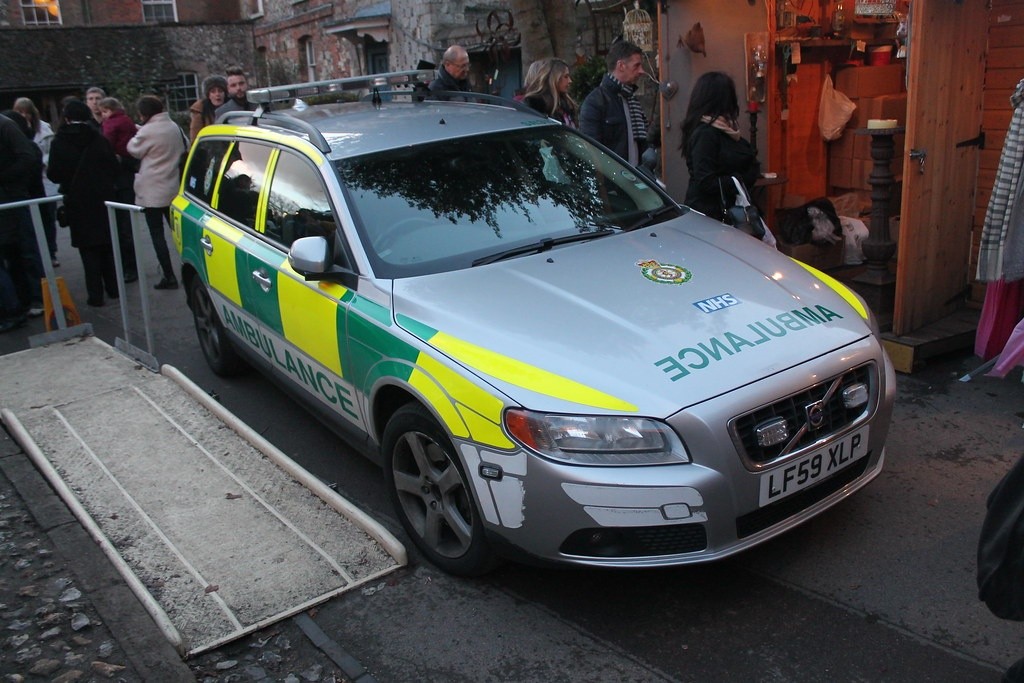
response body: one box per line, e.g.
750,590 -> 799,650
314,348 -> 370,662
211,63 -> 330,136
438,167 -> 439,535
56,206 -> 68,227
819,76 -> 856,142
731,177 -> 777,249
719,176 -> 765,240
782,197 -> 841,244
975,455 -> 1024,621
180,129 -> 187,182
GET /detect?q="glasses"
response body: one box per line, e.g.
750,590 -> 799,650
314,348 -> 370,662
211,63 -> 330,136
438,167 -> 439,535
452,63 -> 472,68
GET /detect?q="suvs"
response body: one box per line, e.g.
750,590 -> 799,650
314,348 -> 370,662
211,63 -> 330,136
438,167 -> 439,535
168,72 -> 896,569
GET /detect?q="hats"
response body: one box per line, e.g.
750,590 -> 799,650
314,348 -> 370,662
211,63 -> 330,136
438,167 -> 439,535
203,75 -> 227,96
65,101 -> 91,120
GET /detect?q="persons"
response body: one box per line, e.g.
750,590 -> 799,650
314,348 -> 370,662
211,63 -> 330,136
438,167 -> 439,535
13,97 -> 61,268
214,65 -> 275,123
3,111 -> 45,317
47,99 -> 120,307
524,58 -> 579,130
86,87 -> 143,282
680,71 -> 777,231
126,95 -> 194,288
585,40 -> 648,169
0,113 -> 36,333
428,43 -> 479,102
513,57 -> 543,100
97,96 -> 137,191
188,74 -> 227,145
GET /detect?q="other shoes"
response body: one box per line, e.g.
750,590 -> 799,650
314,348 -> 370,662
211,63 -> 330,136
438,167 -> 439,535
154,277 -> 178,288
5,314 -> 26,323
52,256 -> 59,266
125,267 -> 138,283
0,318 -> 19,334
28,298 -> 43,317
